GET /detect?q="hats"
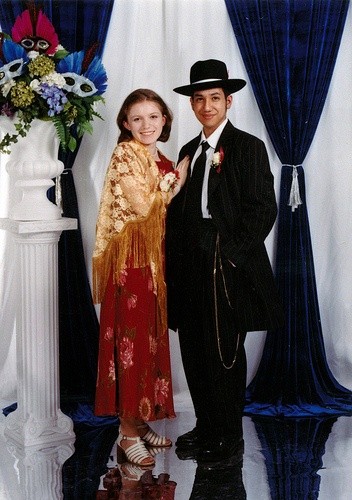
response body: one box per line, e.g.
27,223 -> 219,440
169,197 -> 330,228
173,60 -> 246,96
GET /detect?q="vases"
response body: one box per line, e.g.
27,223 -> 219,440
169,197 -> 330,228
0,114 -> 64,222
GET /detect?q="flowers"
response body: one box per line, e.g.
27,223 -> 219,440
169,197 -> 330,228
208,145 -> 224,174
0,8 -> 106,155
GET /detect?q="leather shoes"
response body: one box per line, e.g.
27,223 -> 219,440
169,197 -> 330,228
198,433 -> 245,461
175,426 -> 206,450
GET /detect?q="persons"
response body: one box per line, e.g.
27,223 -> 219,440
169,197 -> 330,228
163,59 -> 279,472
175,448 -> 247,500
90,87 -> 189,465
103,457 -> 177,500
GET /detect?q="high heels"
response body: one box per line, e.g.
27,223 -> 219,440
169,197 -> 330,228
119,422 -> 172,447
116,433 -> 154,467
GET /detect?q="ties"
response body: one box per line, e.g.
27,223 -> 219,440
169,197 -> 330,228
186,141 -> 211,252
